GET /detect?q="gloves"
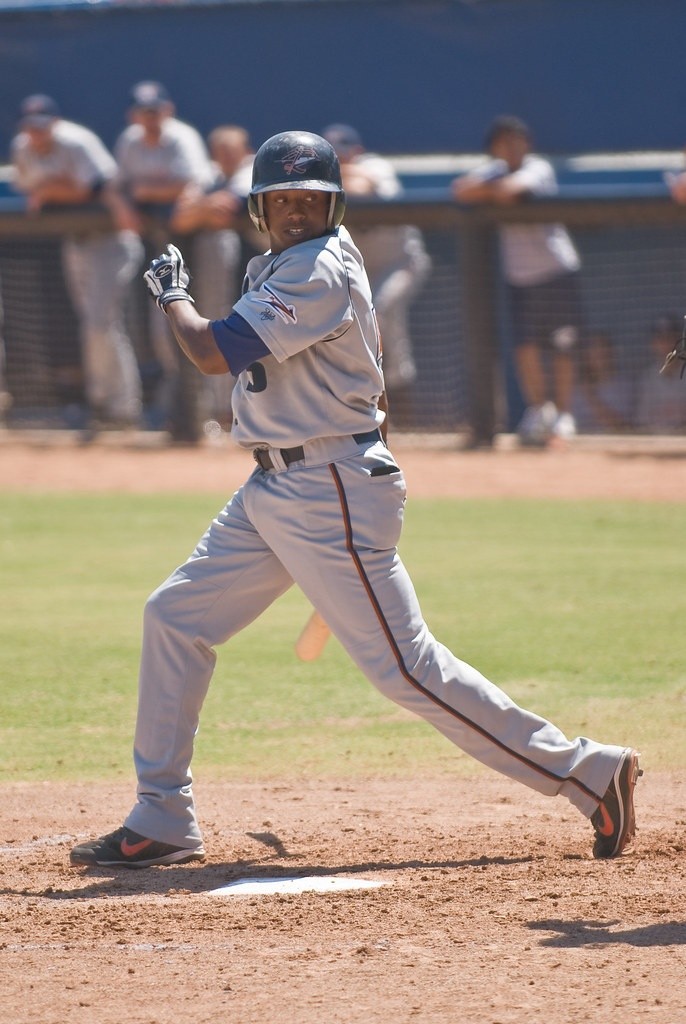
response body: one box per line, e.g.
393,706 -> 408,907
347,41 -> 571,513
143,243 -> 194,316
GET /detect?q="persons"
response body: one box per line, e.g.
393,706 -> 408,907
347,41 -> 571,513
71,132 -> 642,869
10,78 -> 686,452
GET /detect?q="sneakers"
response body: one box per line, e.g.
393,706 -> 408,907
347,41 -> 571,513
70,826 -> 204,869
591,749 -> 642,858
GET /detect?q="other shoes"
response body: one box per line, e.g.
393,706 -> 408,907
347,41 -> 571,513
521,402 -> 578,447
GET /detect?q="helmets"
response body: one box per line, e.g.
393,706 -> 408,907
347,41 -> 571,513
247,131 -> 347,233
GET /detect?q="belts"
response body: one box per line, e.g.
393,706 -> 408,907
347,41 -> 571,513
252,427 -> 381,470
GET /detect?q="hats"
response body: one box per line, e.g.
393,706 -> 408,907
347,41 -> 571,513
130,82 -> 170,116
19,95 -> 59,132
